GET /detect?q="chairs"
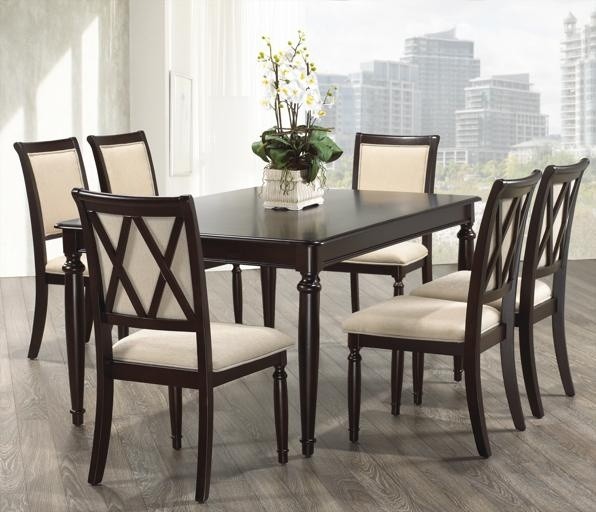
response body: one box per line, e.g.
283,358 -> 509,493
14,131 -> 590,503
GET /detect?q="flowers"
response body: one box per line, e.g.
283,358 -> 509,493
256,31 -> 345,196
